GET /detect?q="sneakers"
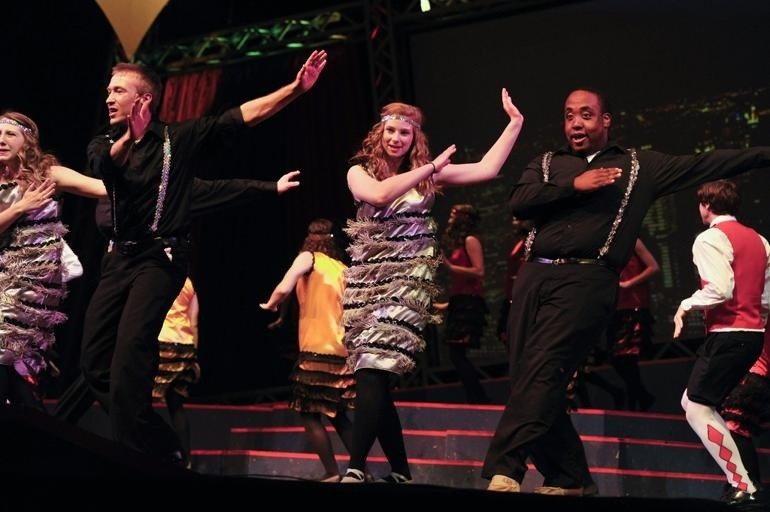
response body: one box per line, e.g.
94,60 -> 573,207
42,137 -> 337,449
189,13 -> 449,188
533,480 -> 599,497
340,468 -> 367,483
486,473 -> 521,492
376,473 -> 414,483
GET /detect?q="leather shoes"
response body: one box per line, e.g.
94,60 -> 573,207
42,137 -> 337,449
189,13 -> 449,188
720,486 -> 763,507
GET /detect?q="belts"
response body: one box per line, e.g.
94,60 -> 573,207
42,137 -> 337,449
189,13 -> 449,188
114,236 -> 177,257
528,257 -> 595,266
